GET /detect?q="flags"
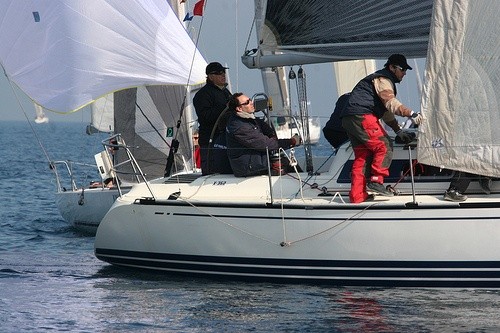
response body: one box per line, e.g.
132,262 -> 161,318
183,12 -> 193,21
194,0 -> 204,16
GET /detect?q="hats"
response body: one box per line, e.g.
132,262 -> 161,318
206,62 -> 229,74
387,54 -> 412,70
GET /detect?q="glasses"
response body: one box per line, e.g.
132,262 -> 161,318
393,65 -> 407,71
236,98 -> 252,107
210,70 -> 225,75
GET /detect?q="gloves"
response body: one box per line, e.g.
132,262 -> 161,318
291,133 -> 301,146
410,113 -> 422,125
398,131 -> 410,143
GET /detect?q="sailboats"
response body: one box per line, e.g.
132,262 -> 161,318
1,0 -> 378,238
93,1 -> 500,289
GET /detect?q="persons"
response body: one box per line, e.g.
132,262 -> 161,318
341,54 -> 422,204
211,132 -> 233,173
226,93 -> 304,177
444,170 -> 472,201
322,93 -> 354,154
193,62 -> 233,176
191,129 -> 201,170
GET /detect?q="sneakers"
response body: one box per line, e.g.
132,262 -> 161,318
367,182 -> 390,195
444,189 -> 467,201
362,197 -> 373,201
478,175 -> 492,194
